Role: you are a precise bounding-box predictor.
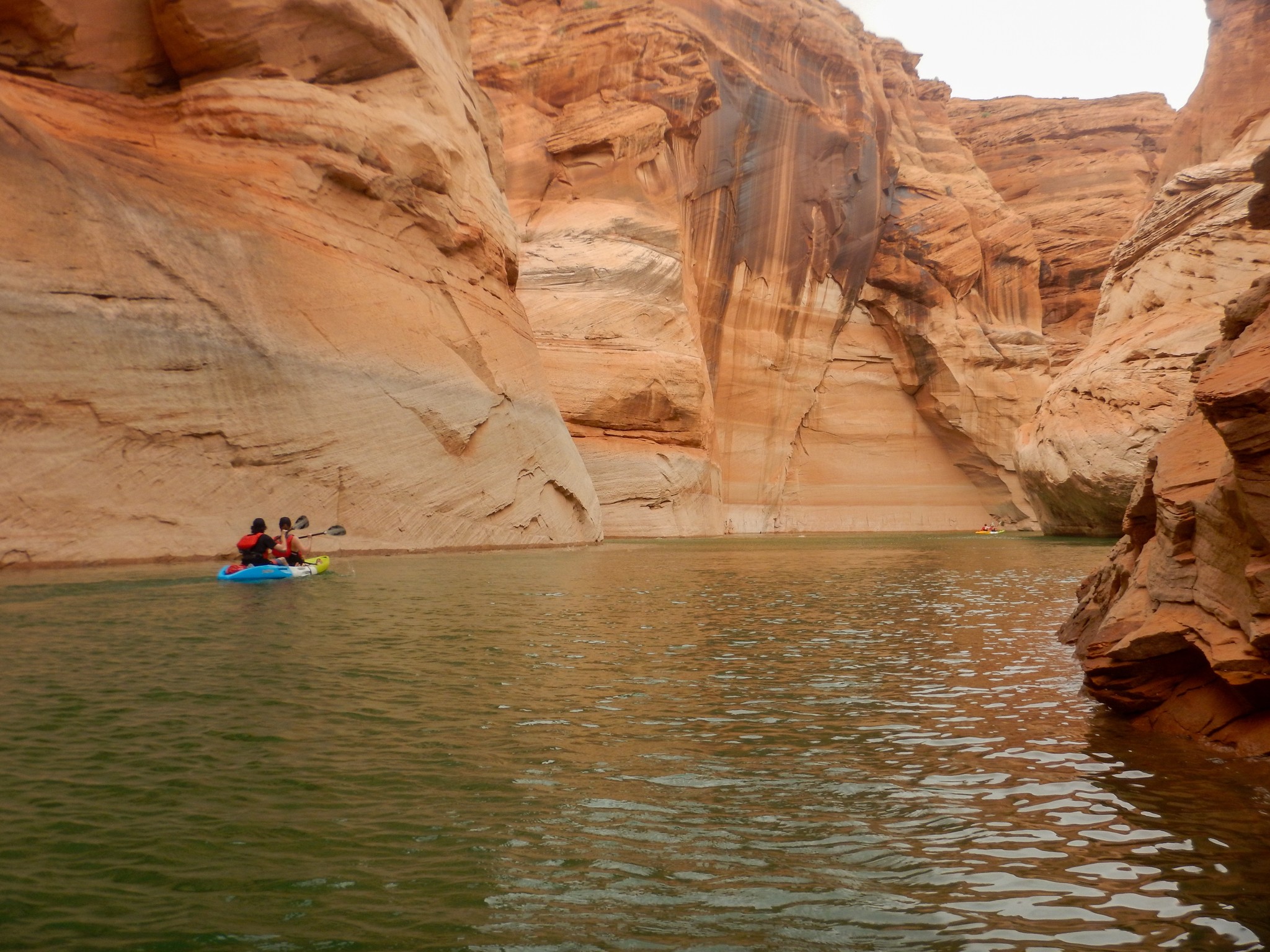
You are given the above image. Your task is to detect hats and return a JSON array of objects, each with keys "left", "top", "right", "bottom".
[
  {"left": 253, "top": 518, "right": 264, "bottom": 527},
  {"left": 280, "top": 517, "right": 291, "bottom": 527}
]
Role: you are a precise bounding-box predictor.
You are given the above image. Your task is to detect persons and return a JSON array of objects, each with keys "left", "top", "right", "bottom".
[
  {"left": 989, "top": 524, "right": 998, "bottom": 532},
  {"left": 981, "top": 524, "right": 991, "bottom": 531},
  {"left": 236, "top": 518, "right": 289, "bottom": 568},
  {"left": 271, "top": 517, "right": 323, "bottom": 567}
]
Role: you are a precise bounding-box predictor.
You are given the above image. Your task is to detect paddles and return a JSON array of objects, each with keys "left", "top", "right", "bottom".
[
  {"left": 298, "top": 525, "right": 346, "bottom": 539},
  {"left": 273, "top": 515, "right": 309, "bottom": 541}
]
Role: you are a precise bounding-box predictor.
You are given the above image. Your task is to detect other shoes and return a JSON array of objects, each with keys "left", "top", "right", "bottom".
[{"left": 316, "top": 558, "right": 323, "bottom": 565}]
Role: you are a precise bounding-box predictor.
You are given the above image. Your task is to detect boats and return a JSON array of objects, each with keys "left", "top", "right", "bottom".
[
  {"left": 214, "top": 553, "right": 336, "bottom": 583},
  {"left": 975, "top": 530, "right": 1005, "bottom": 534}
]
